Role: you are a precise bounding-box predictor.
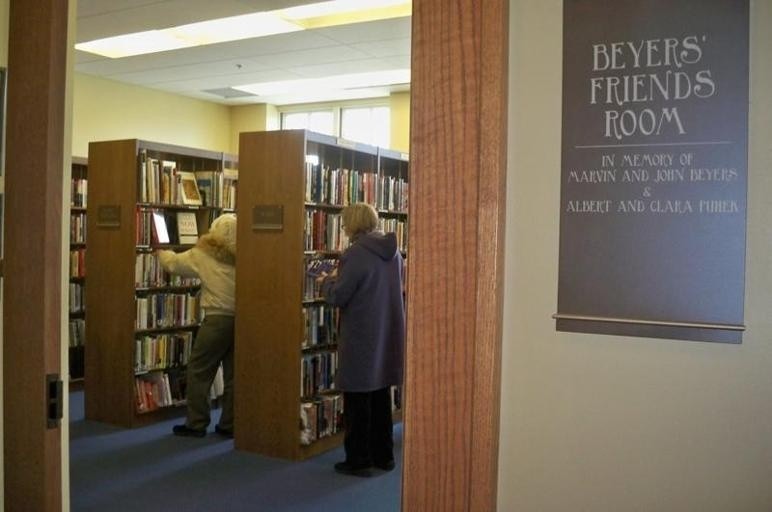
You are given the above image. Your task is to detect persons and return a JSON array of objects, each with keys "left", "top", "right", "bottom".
[
  {"left": 315, "top": 204, "right": 406, "bottom": 473},
  {"left": 155, "top": 213, "right": 238, "bottom": 435}
]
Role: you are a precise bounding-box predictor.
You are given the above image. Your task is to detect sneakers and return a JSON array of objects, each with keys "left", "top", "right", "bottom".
[
  {"left": 215, "top": 424, "right": 233, "bottom": 438},
  {"left": 173, "top": 425, "right": 206, "bottom": 437},
  {"left": 335, "top": 461, "right": 395, "bottom": 477}
]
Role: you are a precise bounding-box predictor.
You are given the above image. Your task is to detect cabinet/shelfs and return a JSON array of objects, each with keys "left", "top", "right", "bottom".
[
  {"left": 69, "top": 156, "right": 88, "bottom": 392},
  {"left": 85, "top": 138, "right": 238, "bottom": 430},
  {"left": 238, "top": 130, "right": 409, "bottom": 461}
]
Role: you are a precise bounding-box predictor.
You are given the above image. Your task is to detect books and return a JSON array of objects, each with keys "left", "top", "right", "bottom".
[
  {"left": 134, "top": 148, "right": 238, "bottom": 411},
  {"left": 306, "top": 163, "right": 409, "bottom": 444},
  {"left": 70, "top": 180, "right": 88, "bottom": 347}
]
